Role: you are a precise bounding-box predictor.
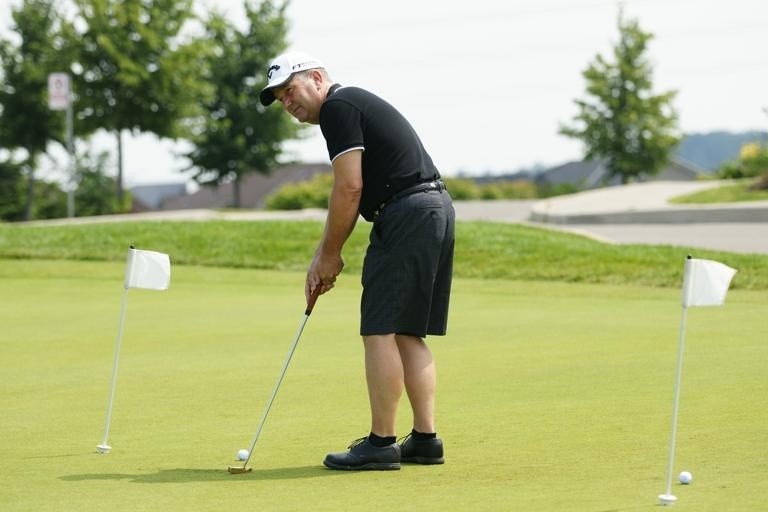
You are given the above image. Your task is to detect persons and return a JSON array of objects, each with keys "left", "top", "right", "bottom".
[{"left": 260, "top": 52, "right": 455, "bottom": 470}]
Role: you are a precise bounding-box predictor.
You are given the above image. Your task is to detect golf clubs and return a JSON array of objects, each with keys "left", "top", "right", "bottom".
[{"left": 228, "top": 275, "right": 323, "bottom": 473}]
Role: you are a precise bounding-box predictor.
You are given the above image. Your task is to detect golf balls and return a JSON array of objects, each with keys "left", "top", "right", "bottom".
[
  {"left": 680, "top": 471, "right": 691, "bottom": 483},
  {"left": 237, "top": 449, "right": 248, "bottom": 460}
]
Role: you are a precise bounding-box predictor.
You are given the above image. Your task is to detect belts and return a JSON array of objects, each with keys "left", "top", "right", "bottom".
[{"left": 398, "top": 182, "right": 445, "bottom": 197}]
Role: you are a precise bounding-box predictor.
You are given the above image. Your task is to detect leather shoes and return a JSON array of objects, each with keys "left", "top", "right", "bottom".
[{"left": 324, "top": 433, "right": 444, "bottom": 469}]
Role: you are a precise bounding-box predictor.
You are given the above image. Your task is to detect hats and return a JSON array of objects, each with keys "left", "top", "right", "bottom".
[{"left": 260, "top": 53, "right": 323, "bottom": 106}]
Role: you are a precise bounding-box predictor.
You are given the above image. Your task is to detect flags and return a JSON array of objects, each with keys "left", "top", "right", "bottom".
[
  {"left": 127, "top": 249, "right": 170, "bottom": 290},
  {"left": 684, "top": 260, "right": 738, "bottom": 307}
]
